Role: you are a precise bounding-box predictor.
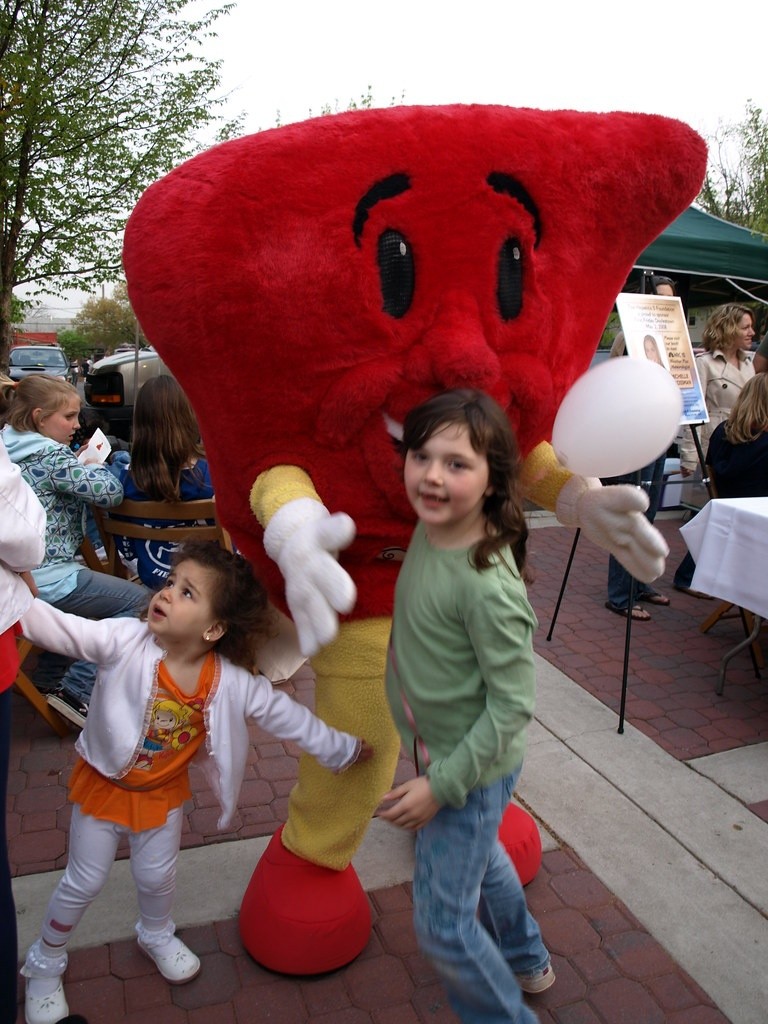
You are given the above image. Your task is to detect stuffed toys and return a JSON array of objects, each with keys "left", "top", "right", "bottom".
[{"left": 124, "top": 105, "right": 709, "bottom": 972}]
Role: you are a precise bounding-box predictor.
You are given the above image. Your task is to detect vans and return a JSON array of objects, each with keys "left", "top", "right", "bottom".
[{"left": 77, "top": 340, "right": 174, "bottom": 442}]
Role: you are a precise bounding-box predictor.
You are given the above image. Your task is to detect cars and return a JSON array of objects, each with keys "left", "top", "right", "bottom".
[{"left": 8, "top": 345, "right": 73, "bottom": 386}]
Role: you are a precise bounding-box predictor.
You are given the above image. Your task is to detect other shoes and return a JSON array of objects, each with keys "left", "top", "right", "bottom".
[
  {"left": 19, "top": 962, "right": 69, "bottom": 1024},
  {"left": 46, "top": 685, "right": 89, "bottom": 728},
  {"left": 137, "top": 932, "right": 201, "bottom": 984},
  {"left": 672, "top": 585, "right": 716, "bottom": 600},
  {"left": 514, "top": 960, "right": 555, "bottom": 993}
]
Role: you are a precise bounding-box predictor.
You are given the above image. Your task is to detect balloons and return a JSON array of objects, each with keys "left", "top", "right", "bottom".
[{"left": 551, "top": 356, "right": 684, "bottom": 478}]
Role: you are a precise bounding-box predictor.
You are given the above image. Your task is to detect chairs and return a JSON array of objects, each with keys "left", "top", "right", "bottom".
[
  {"left": 701, "top": 601, "right": 763, "bottom": 678},
  {"left": 20, "top": 356, "right": 31, "bottom": 362},
  {"left": 49, "top": 356, "right": 59, "bottom": 363},
  {"left": 12, "top": 497, "right": 234, "bottom": 739}
]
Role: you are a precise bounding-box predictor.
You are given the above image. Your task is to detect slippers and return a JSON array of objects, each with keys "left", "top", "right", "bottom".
[
  {"left": 605, "top": 600, "right": 651, "bottom": 621},
  {"left": 637, "top": 592, "right": 670, "bottom": 605}
]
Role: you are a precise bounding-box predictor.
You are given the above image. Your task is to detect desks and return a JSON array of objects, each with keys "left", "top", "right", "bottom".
[{"left": 675, "top": 497, "right": 768, "bottom": 702}]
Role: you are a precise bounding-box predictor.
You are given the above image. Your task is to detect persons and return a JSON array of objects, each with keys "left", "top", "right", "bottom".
[
  {"left": 0, "top": 435, "right": 86, "bottom": 1024},
  {"left": 19, "top": 541, "right": 373, "bottom": 1024},
  {"left": 604, "top": 276, "right": 675, "bottom": 619},
  {"left": 71, "top": 374, "right": 242, "bottom": 592},
  {"left": 378, "top": 388, "right": 556, "bottom": 1024},
  {"left": 704, "top": 332, "right": 768, "bottom": 498},
  {"left": 672, "top": 303, "right": 756, "bottom": 598},
  {"left": 0, "top": 373, "right": 151, "bottom": 729},
  {"left": 644, "top": 335, "right": 664, "bottom": 367},
  {"left": 72, "top": 358, "right": 93, "bottom": 385}
]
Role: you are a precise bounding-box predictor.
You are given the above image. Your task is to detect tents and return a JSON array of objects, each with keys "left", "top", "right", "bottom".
[{"left": 612, "top": 207, "right": 768, "bottom": 309}]
